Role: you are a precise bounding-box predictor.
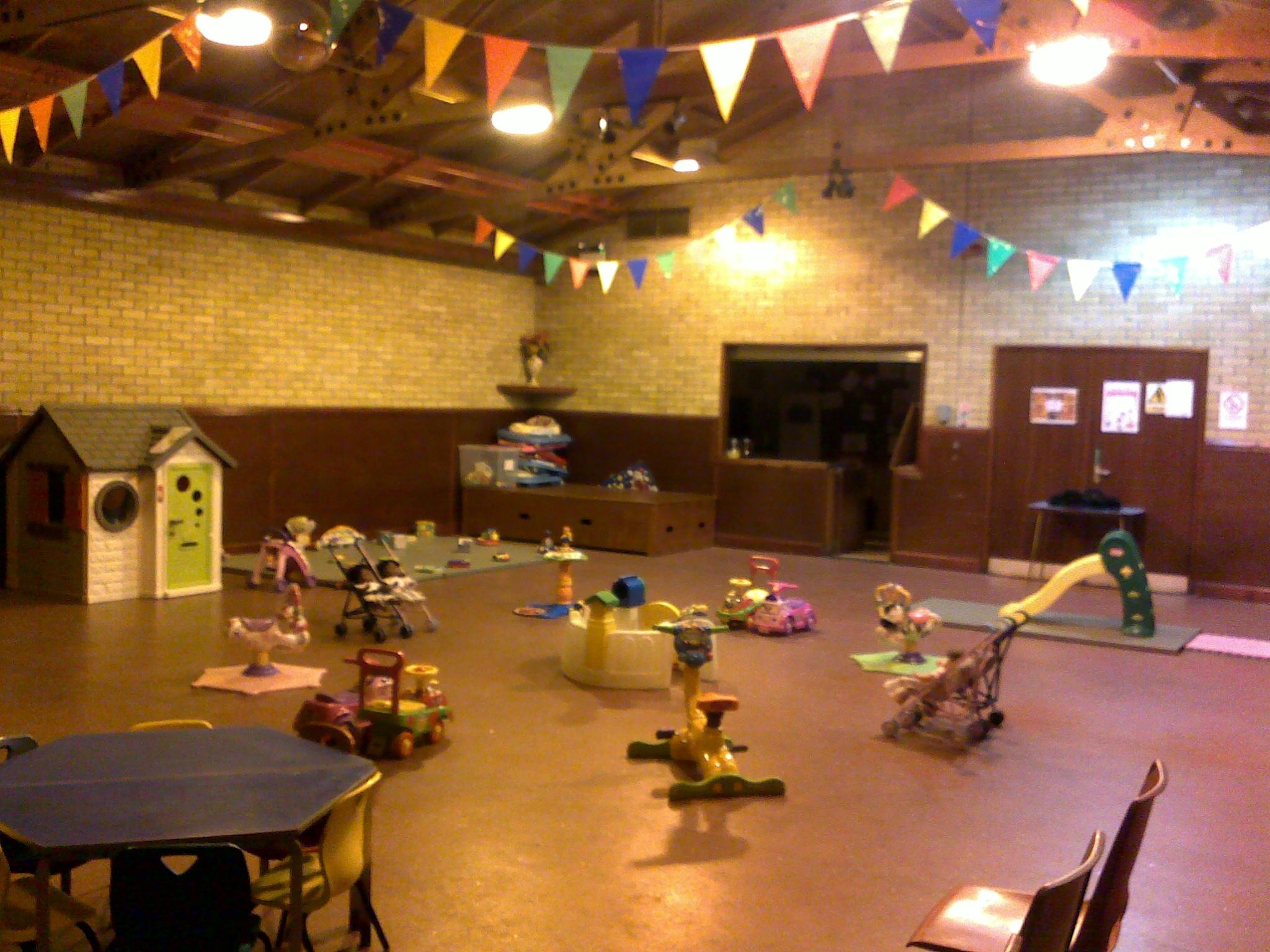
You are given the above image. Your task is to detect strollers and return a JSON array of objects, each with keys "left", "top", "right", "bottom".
[
  {"left": 879, "top": 611, "right": 1030, "bottom": 739},
  {"left": 328, "top": 529, "right": 439, "bottom": 643}
]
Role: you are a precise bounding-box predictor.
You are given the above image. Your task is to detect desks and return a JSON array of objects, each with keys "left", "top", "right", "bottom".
[{"left": 0, "top": 729, "right": 374, "bottom": 952}]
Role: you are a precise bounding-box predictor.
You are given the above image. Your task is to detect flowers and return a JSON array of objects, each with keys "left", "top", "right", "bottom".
[{"left": 520, "top": 328, "right": 553, "bottom": 361}]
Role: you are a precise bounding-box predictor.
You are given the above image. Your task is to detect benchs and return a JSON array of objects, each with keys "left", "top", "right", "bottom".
[
  {"left": 463, "top": 484, "right": 715, "bottom": 557},
  {"left": 1029, "top": 502, "right": 1146, "bottom": 556}
]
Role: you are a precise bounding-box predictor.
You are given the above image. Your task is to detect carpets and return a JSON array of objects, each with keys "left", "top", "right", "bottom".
[{"left": 223, "top": 536, "right": 547, "bottom": 582}]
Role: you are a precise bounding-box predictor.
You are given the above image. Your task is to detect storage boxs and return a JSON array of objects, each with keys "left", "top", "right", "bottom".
[
  {"left": 460, "top": 444, "right": 521, "bottom": 488},
  {"left": 561, "top": 620, "right": 674, "bottom": 690}
]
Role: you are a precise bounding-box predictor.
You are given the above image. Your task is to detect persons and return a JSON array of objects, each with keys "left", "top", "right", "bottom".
[
  {"left": 885, "top": 650, "right": 964, "bottom": 704},
  {"left": 338, "top": 560, "right": 423, "bottom": 603}
]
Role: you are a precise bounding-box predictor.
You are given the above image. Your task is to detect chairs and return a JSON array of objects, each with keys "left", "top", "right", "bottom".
[
  {"left": 906, "top": 759, "right": 1166, "bottom": 952},
  {"left": 0, "top": 720, "right": 390, "bottom": 952}
]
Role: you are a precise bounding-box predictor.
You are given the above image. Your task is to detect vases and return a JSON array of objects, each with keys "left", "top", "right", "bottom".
[{"left": 527, "top": 347, "right": 543, "bottom": 387}]
